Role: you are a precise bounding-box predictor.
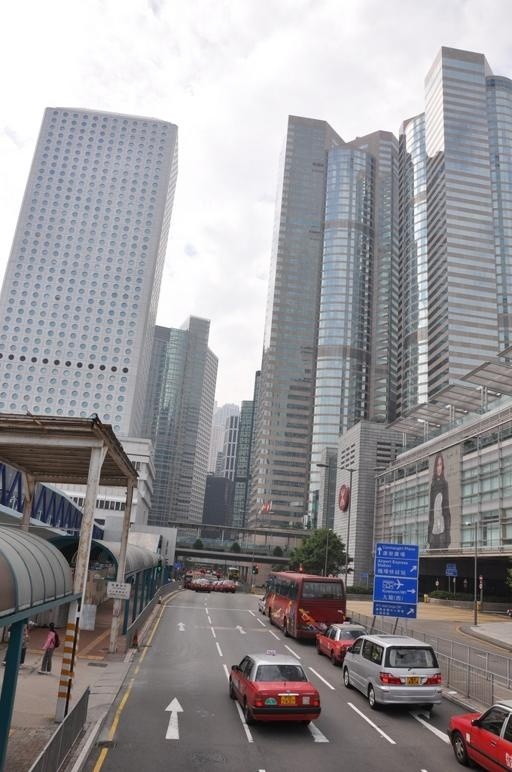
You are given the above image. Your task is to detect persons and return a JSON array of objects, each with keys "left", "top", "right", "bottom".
[
  {"left": 37, "top": 627, "right": 56, "bottom": 675},
  {"left": 19, "top": 628, "right": 29, "bottom": 668},
  {"left": 427, "top": 452, "right": 451, "bottom": 552}
]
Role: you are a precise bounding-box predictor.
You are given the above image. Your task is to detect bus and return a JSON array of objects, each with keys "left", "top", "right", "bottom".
[{"left": 264, "top": 570, "right": 347, "bottom": 641}]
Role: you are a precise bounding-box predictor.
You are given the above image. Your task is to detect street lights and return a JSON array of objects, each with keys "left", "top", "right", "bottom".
[{"left": 315, "top": 463, "right": 357, "bottom": 574}]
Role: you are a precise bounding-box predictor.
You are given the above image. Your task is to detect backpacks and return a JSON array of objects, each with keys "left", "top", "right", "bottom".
[{"left": 52, "top": 630, "right": 59, "bottom": 647}]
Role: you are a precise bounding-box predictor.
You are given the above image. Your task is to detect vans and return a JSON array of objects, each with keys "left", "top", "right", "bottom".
[{"left": 341, "top": 633, "right": 443, "bottom": 710}]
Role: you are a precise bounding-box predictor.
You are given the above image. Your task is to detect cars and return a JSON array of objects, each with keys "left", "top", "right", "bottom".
[
  {"left": 229, "top": 648, "right": 322, "bottom": 727},
  {"left": 446, "top": 699, "right": 512, "bottom": 772},
  {"left": 315, "top": 621, "right": 369, "bottom": 667}
]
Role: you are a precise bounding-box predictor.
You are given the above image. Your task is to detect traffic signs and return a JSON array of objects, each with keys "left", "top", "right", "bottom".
[{"left": 372, "top": 542, "right": 420, "bottom": 619}]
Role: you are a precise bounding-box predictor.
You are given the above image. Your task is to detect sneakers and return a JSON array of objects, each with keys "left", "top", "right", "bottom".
[{"left": 2, "top": 661, "right": 52, "bottom": 675}]
